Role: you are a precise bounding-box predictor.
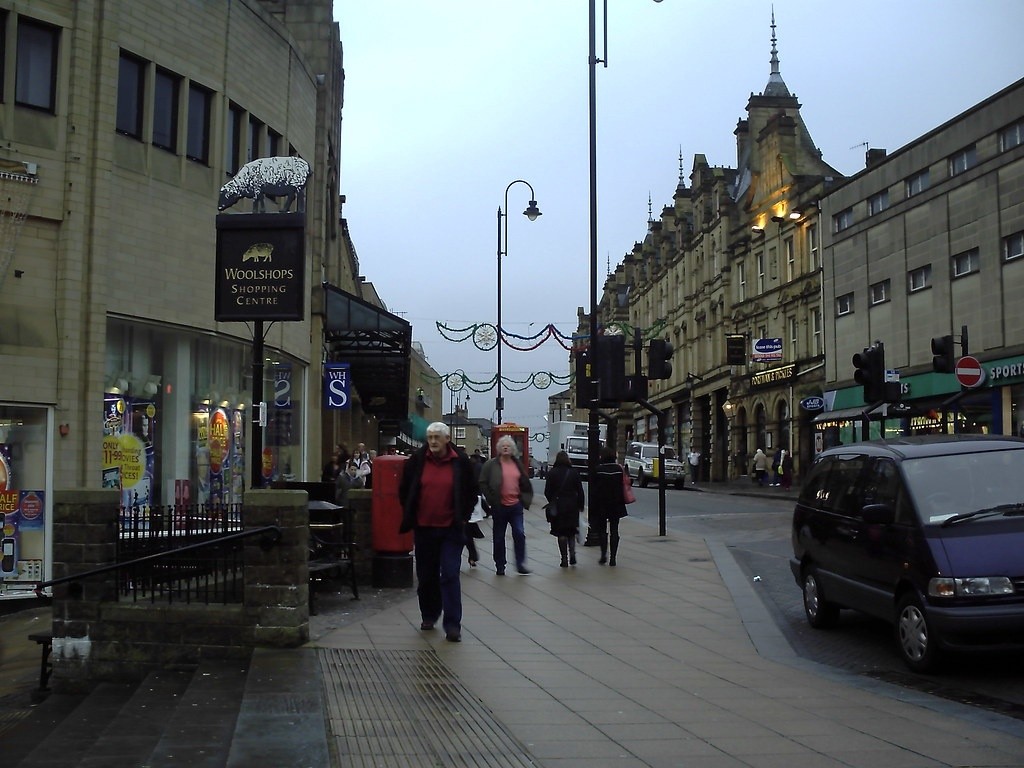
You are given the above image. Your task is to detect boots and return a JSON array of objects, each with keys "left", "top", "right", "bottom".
[
  {"left": 481, "top": 499, "right": 492, "bottom": 518},
  {"left": 559, "top": 539, "right": 568, "bottom": 567},
  {"left": 609, "top": 535, "right": 621, "bottom": 566},
  {"left": 598, "top": 532, "right": 608, "bottom": 564}
]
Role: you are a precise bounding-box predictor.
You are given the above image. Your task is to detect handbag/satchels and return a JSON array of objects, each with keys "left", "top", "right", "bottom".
[
  {"left": 752, "top": 463, "right": 756, "bottom": 473},
  {"left": 618, "top": 463, "right": 636, "bottom": 504},
  {"left": 542, "top": 502, "right": 558, "bottom": 523},
  {"left": 778, "top": 466, "right": 783, "bottom": 475}
]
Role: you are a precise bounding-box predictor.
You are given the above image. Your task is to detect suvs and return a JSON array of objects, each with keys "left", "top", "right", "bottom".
[{"left": 624, "top": 441, "right": 686, "bottom": 490}]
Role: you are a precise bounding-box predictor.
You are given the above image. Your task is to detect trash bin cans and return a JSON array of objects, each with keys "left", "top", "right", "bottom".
[
  {"left": 271, "top": 481, "right": 337, "bottom": 505},
  {"left": 369, "top": 455, "right": 414, "bottom": 588}
]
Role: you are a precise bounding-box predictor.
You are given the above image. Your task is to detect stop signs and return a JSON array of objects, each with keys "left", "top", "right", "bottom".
[{"left": 955, "top": 356, "right": 982, "bottom": 387}]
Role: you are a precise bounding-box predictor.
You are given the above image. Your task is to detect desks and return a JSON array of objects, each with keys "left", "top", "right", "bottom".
[{"left": 309, "top": 520, "right": 344, "bottom": 530}]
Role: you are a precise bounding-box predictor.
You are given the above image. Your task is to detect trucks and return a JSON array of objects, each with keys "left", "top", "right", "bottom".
[{"left": 547, "top": 420, "right": 607, "bottom": 476}]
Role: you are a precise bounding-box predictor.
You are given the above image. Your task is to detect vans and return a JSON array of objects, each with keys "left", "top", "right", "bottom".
[{"left": 789, "top": 433, "right": 1024, "bottom": 673}]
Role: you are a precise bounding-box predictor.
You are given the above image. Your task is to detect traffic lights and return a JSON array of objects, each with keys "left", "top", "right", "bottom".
[
  {"left": 852, "top": 353, "right": 869, "bottom": 384},
  {"left": 931, "top": 337, "right": 946, "bottom": 369},
  {"left": 664, "top": 342, "right": 674, "bottom": 379}
]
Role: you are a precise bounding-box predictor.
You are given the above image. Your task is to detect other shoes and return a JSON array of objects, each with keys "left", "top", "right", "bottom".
[
  {"left": 769, "top": 483, "right": 776, "bottom": 486},
  {"left": 568, "top": 536, "right": 576, "bottom": 564},
  {"left": 776, "top": 483, "right": 780, "bottom": 486},
  {"left": 496, "top": 568, "right": 505, "bottom": 575},
  {"left": 517, "top": 567, "right": 534, "bottom": 575},
  {"left": 420, "top": 619, "right": 436, "bottom": 629},
  {"left": 446, "top": 627, "right": 461, "bottom": 642}
]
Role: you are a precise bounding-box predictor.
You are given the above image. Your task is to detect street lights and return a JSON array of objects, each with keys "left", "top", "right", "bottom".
[
  {"left": 456, "top": 388, "right": 471, "bottom": 448},
  {"left": 496, "top": 179, "right": 543, "bottom": 426}
]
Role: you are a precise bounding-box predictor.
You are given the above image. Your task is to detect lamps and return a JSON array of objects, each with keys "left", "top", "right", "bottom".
[
  {"left": 142, "top": 374, "right": 162, "bottom": 396},
  {"left": 751, "top": 225, "right": 764, "bottom": 234},
  {"left": 415, "top": 384, "right": 425, "bottom": 400},
  {"left": 722, "top": 241, "right": 748, "bottom": 255},
  {"left": 771, "top": 215, "right": 785, "bottom": 223},
  {"left": 106, "top": 373, "right": 132, "bottom": 391},
  {"left": 725, "top": 402, "right": 734, "bottom": 410},
  {"left": 200, "top": 397, "right": 213, "bottom": 405},
  {"left": 789, "top": 209, "right": 804, "bottom": 220},
  {"left": 221, "top": 399, "right": 245, "bottom": 410}
]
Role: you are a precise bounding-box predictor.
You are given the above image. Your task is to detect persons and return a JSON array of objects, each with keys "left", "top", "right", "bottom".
[
  {"left": 688, "top": 446, "right": 701, "bottom": 485},
  {"left": 781, "top": 450, "right": 796, "bottom": 491},
  {"left": 399, "top": 421, "right": 478, "bottom": 641},
  {"left": 769, "top": 446, "right": 782, "bottom": 487},
  {"left": 539, "top": 451, "right": 585, "bottom": 568},
  {"left": 479, "top": 433, "right": 534, "bottom": 578},
  {"left": 592, "top": 446, "right": 635, "bottom": 567},
  {"left": 753, "top": 448, "right": 768, "bottom": 487},
  {"left": 328, "top": 431, "right": 497, "bottom": 527}
]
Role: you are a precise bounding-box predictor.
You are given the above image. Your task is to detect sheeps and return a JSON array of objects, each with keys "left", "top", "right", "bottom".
[{"left": 217, "top": 156, "right": 311, "bottom": 214}]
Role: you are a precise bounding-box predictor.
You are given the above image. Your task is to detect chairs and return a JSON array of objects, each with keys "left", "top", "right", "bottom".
[{"left": 311, "top": 504, "right": 361, "bottom": 617}]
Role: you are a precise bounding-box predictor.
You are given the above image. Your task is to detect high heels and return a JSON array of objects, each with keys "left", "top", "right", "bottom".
[{"left": 468, "top": 558, "right": 475, "bottom": 568}]
